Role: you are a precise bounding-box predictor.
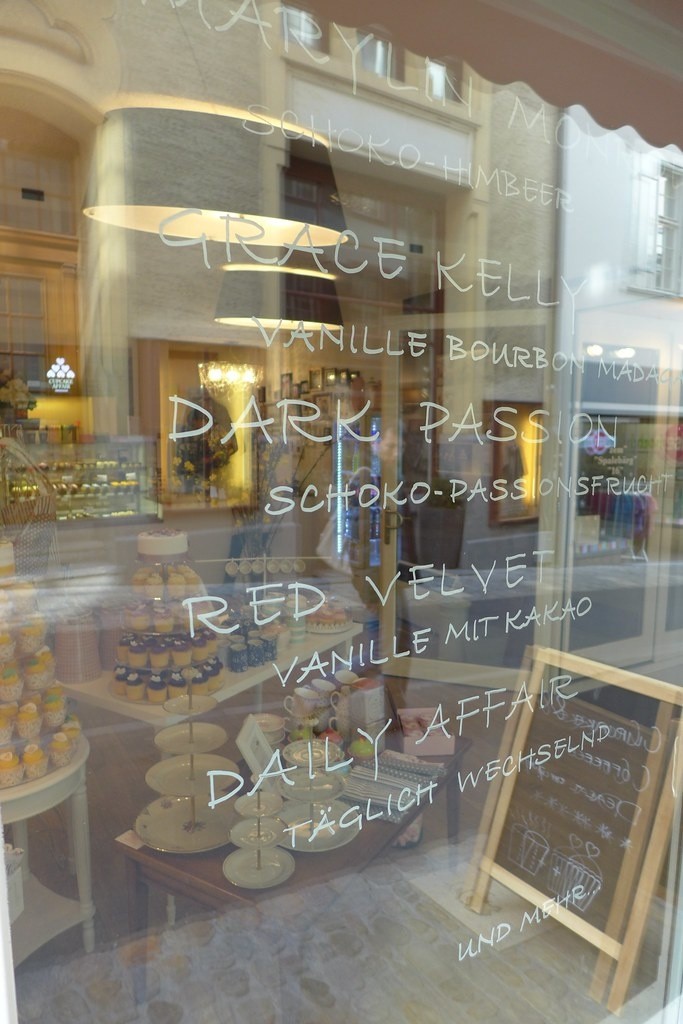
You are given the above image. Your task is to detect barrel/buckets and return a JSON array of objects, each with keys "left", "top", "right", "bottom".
[
  {"left": 244, "top": 713, "right": 284, "bottom": 755},
  {"left": 53, "top": 599, "right": 126, "bottom": 684}
]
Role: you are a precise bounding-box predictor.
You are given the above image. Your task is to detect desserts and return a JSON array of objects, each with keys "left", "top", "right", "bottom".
[
  {"left": 11, "top": 458, "right": 142, "bottom": 519},
  {"left": 0, "top": 542, "right": 81, "bottom": 787},
  {"left": 112, "top": 529, "right": 225, "bottom": 702}
]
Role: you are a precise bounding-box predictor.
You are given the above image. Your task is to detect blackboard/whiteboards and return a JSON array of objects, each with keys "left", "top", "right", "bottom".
[{"left": 484, "top": 685, "right": 654, "bottom": 938}]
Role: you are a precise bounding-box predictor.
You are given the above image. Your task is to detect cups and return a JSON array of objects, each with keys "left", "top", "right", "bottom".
[
  {"left": 261, "top": 592, "right": 306, "bottom": 644},
  {"left": 346, "top": 736, "right": 377, "bottom": 765},
  {"left": 284, "top": 669, "right": 359, "bottom": 733},
  {"left": 317, "top": 728, "right": 343, "bottom": 750},
  {"left": 286, "top": 724, "right": 315, "bottom": 745},
  {"left": 258, "top": 620, "right": 290, "bottom": 652},
  {"left": 224, "top": 630, "right": 278, "bottom": 672}
]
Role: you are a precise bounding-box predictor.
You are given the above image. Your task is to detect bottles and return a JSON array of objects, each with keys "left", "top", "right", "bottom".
[{"left": 344, "top": 421, "right": 380, "bottom": 558}]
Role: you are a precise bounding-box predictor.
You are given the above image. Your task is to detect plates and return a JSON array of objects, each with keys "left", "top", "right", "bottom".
[
  {"left": 234, "top": 791, "right": 283, "bottom": 818},
  {"left": 145, "top": 754, "right": 240, "bottom": 797},
  {"left": 271, "top": 800, "right": 360, "bottom": 853},
  {"left": 222, "top": 847, "right": 296, "bottom": 889},
  {"left": 163, "top": 695, "right": 219, "bottom": 717},
  {"left": 226, "top": 818, "right": 290, "bottom": 851},
  {"left": 277, "top": 768, "right": 346, "bottom": 805},
  {"left": 153, "top": 722, "right": 228, "bottom": 756},
  {"left": 134, "top": 791, "right": 243, "bottom": 853},
  {"left": 282, "top": 739, "right": 342, "bottom": 767}
]
